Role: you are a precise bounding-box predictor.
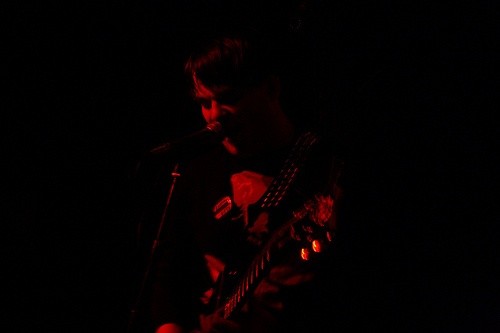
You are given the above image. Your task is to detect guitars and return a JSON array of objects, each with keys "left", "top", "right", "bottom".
[{"left": 223, "top": 195, "right": 333, "bottom": 320}]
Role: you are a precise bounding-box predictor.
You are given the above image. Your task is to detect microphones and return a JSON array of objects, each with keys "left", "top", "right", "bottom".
[{"left": 152, "top": 121, "right": 224, "bottom": 152}]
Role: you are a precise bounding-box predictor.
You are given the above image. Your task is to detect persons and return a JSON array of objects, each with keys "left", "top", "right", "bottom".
[{"left": 146, "top": 39, "right": 372, "bottom": 333}]
